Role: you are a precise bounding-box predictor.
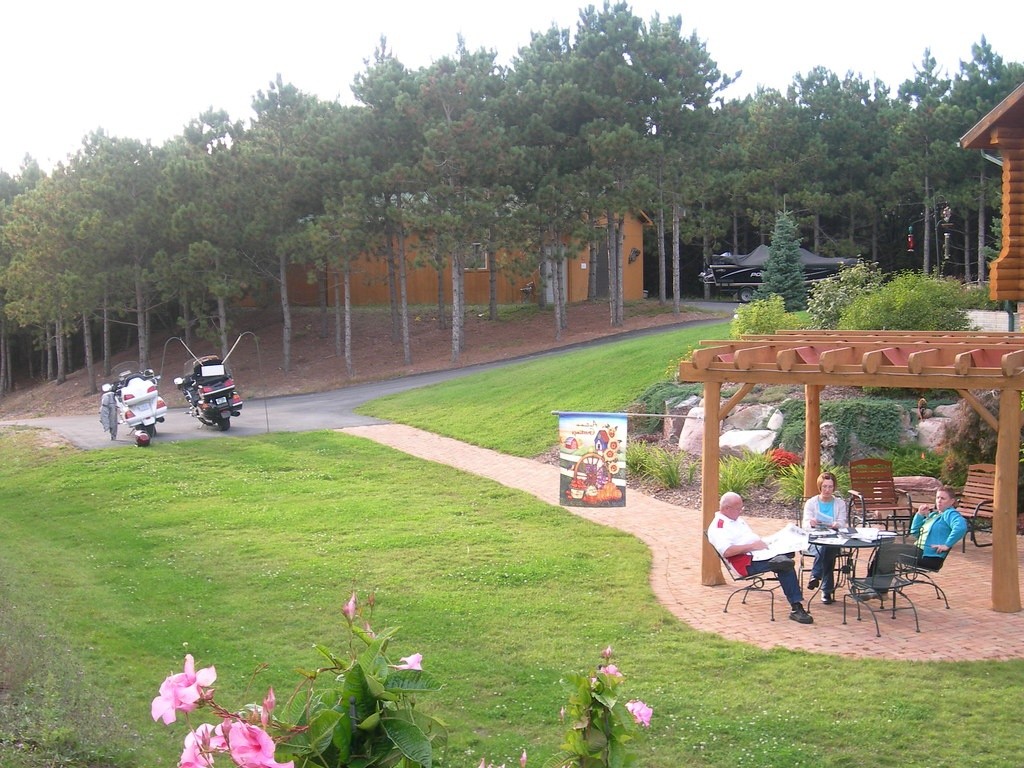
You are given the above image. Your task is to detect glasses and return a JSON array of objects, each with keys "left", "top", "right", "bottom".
[{"left": 729, "top": 505, "right": 746, "bottom": 511}]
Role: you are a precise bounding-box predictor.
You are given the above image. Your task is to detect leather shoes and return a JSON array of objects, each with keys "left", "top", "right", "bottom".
[
  {"left": 789, "top": 604, "right": 814, "bottom": 623},
  {"left": 768, "top": 554, "right": 795, "bottom": 573}
]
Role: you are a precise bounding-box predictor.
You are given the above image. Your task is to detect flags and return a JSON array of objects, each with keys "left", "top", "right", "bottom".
[{"left": 559, "top": 413, "right": 626, "bottom": 508}]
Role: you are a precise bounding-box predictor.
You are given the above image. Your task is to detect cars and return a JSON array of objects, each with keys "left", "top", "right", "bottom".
[{"left": 698, "top": 243, "right": 867, "bottom": 303}]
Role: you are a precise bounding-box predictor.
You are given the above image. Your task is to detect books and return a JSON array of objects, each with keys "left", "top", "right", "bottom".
[{"left": 846, "top": 528, "right": 858, "bottom": 536}]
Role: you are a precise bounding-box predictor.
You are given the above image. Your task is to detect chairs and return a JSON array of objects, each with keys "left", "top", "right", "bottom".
[
  {"left": 848, "top": 458, "right": 912, "bottom": 538},
  {"left": 953, "top": 463, "right": 995, "bottom": 553},
  {"left": 842, "top": 527, "right": 924, "bottom": 636},
  {"left": 794, "top": 493, "right": 851, "bottom": 597},
  {"left": 703, "top": 529, "right": 782, "bottom": 621},
  {"left": 895, "top": 544, "right": 955, "bottom": 609}
]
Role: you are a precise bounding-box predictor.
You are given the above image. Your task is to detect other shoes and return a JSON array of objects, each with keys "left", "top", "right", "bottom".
[
  {"left": 851, "top": 582, "right": 873, "bottom": 590},
  {"left": 856, "top": 588, "right": 888, "bottom": 601},
  {"left": 807, "top": 575, "right": 819, "bottom": 590},
  {"left": 821, "top": 590, "right": 832, "bottom": 604}
]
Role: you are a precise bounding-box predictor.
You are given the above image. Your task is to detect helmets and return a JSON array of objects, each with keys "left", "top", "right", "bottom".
[{"left": 134, "top": 429, "right": 151, "bottom": 447}]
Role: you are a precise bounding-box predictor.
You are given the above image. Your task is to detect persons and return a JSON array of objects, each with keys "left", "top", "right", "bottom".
[
  {"left": 707, "top": 491, "right": 814, "bottom": 624},
  {"left": 802, "top": 472, "right": 846, "bottom": 604},
  {"left": 859, "top": 485, "right": 967, "bottom": 601}
]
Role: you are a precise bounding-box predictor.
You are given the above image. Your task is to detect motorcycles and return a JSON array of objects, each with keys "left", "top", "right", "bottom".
[
  {"left": 174, "top": 355, "right": 243, "bottom": 432},
  {"left": 102, "top": 361, "right": 168, "bottom": 438}
]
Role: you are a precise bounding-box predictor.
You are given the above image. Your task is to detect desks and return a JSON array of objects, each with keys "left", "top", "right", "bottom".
[{"left": 803, "top": 528, "right": 896, "bottom": 612}]
